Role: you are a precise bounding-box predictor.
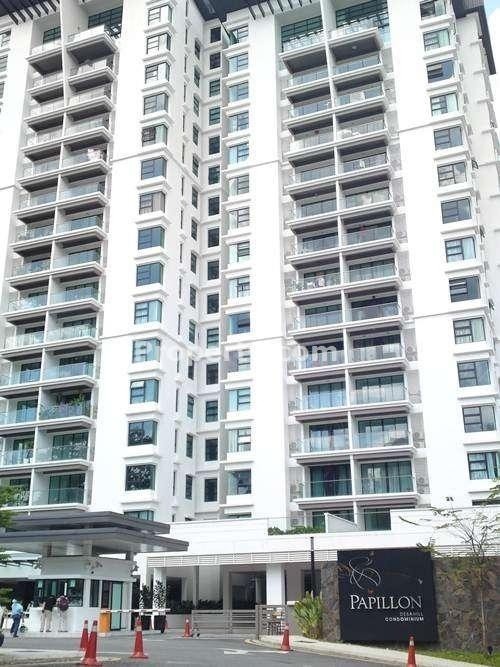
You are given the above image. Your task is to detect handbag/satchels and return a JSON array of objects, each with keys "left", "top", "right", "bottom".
[
  {"left": 61, "top": 604, "right": 68, "bottom": 611},
  {"left": 20, "top": 624, "right": 25, "bottom": 632}
]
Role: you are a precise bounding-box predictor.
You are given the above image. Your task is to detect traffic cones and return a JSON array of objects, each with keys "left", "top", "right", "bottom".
[
  {"left": 406, "top": 636, "right": 418, "bottom": 667},
  {"left": 279, "top": 623, "right": 294, "bottom": 651},
  {"left": 78, "top": 620, "right": 104, "bottom": 667},
  {"left": 181, "top": 618, "right": 192, "bottom": 637},
  {"left": 128, "top": 618, "right": 148, "bottom": 658}
]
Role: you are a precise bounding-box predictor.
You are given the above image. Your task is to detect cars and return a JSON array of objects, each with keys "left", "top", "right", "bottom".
[{"left": 3, "top": 612, "right": 29, "bottom": 633}]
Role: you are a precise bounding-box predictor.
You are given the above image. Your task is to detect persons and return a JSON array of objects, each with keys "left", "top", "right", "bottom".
[
  {"left": 10, "top": 598, "right": 24, "bottom": 640},
  {"left": 39, "top": 592, "right": 71, "bottom": 633}
]
